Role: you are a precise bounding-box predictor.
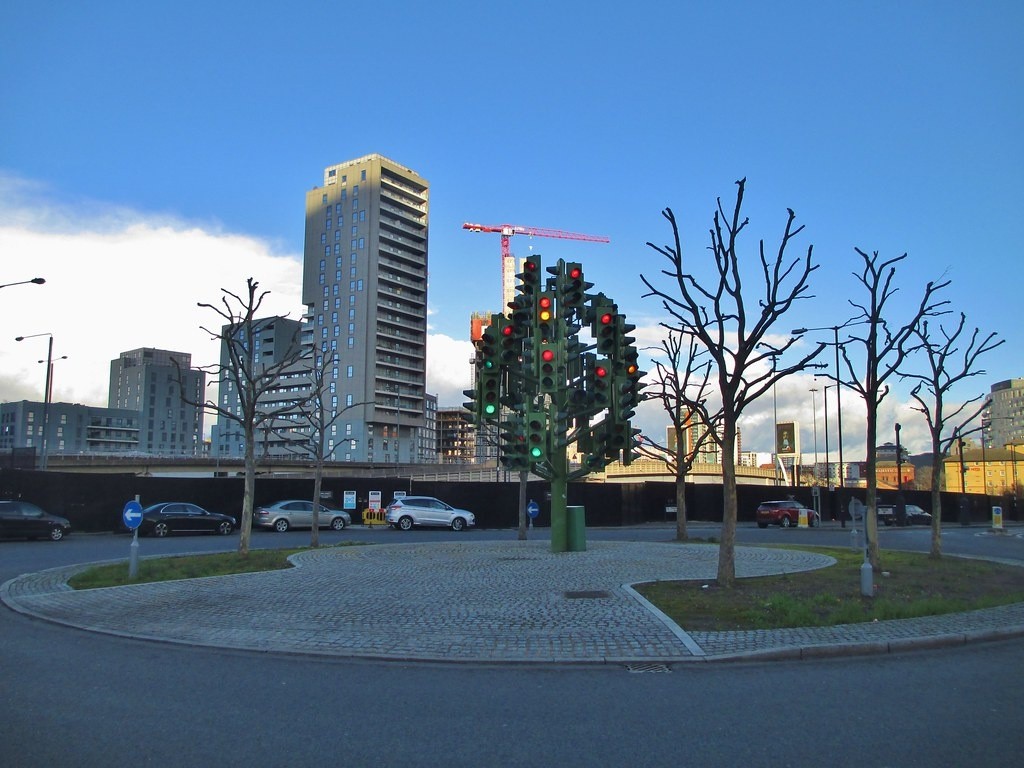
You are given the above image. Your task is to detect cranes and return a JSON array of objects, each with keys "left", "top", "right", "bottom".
[{"left": 461, "top": 221, "right": 610, "bottom": 318}]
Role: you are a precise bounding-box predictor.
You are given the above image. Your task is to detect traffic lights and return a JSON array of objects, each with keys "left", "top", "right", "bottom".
[
  {"left": 595, "top": 306, "right": 614, "bottom": 355},
  {"left": 482, "top": 370, "right": 499, "bottom": 420},
  {"left": 538, "top": 343, "right": 559, "bottom": 393},
  {"left": 613, "top": 313, "right": 637, "bottom": 364},
  {"left": 594, "top": 359, "right": 613, "bottom": 408},
  {"left": 507, "top": 253, "right": 541, "bottom": 328},
  {"left": 481, "top": 327, "right": 500, "bottom": 373},
  {"left": 500, "top": 395, "right": 531, "bottom": 471},
  {"left": 528, "top": 412, "right": 547, "bottom": 463},
  {"left": 622, "top": 420, "right": 642, "bottom": 467},
  {"left": 623, "top": 351, "right": 648, "bottom": 408},
  {"left": 581, "top": 414, "right": 629, "bottom": 473},
  {"left": 520, "top": 327, "right": 543, "bottom": 378},
  {"left": 565, "top": 262, "right": 583, "bottom": 307},
  {"left": 615, "top": 375, "right": 636, "bottom": 424},
  {"left": 576, "top": 272, "right": 596, "bottom": 319},
  {"left": 545, "top": 258, "right": 578, "bottom": 319},
  {"left": 462, "top": 381, "right": 483, "bottom": 429},
  {"left": 567, "top": 387, "right": 588, "bottom": 407},
  {"left": 550, "top": 403, "right": 570, "bottom": 455},
  {"left": 536, "top": 291, "right": 554, "bottom": 343},
  {"left": 599, "top": 297, "right": 618, "bottom": 312},
  {"left": 567, "top": 335, "right": 588, "bottom": 380},
  {"left": 498, "top": 318, "right": 516, "bottom": 365},
  {"left": 557, "top": 316, "right": 579, "bottom": 369}
]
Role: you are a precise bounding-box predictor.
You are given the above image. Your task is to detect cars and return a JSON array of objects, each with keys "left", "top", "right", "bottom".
[
  {"left": 0, "top": 501, "right": 72, "bottom": 541},
  {"left": 883, "top": 504, "right": 933, "bottom": 527},
  {"left": 252, "top": 500, "right": 352, "bottom": 532},
  {"left": 119, "top": 501, "right": 237, "bottom": 539}
]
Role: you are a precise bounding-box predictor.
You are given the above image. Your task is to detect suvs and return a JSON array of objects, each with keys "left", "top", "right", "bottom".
[
  {"left": 384, "top": 496, "right": 476, "bottom": 531},
  {"left": 756, "top": 501, "right": 820, "bottom": 529}
]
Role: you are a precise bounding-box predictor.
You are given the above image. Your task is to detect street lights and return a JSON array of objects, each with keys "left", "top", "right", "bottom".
[
  {"left": 15, "top": 332, "right": 68, "bottom": 470},
  {"left": 791, "top": 317, "right": 886, "bottom": 528},
  {"left": 808, "top": 388, "right": 818, "bottom": 528},
  {"left": 824, "top": 379, "right": 856, "bottom": 522}
]
